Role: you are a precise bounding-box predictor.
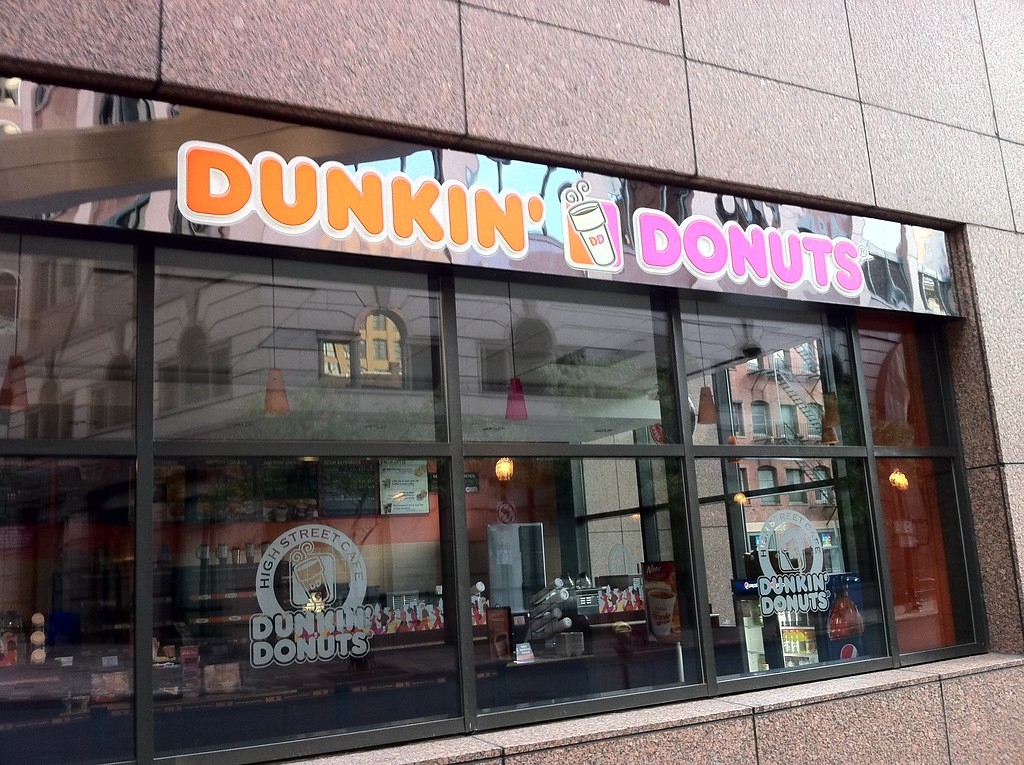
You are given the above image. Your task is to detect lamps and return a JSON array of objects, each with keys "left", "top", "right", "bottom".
[
  {"left": 695, "top": 300, "right": 719, "bottom": 424},
  {"left": 726, "top": 369, "right": 744, "bottom": 464},
  {"left": 504, "top": 282, "right": 528, "bottom": 422},
  {"left": 819, "top": 313, "right": 842, "bottom": 445},
  {"left": 0, "top": 235, "right": 29, "bottom": 410},
  {"left": 263, "top": 259, "right": 290, "bottom": 418}
]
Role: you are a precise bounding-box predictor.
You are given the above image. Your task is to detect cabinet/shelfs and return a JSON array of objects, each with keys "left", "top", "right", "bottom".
[{"left": 54, "top": 560, "right": 293, "bottom": 650}]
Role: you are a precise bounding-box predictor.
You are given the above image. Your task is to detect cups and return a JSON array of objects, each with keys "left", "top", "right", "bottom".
[
  {"left": 194, "top": 540, "right": 274, "bottom": 568},
  {"left": 647, "top": 589, "right": 676, "bottom": 638},
  {"left": 28, "top": 613, "right": 46, "bottom": 664}
]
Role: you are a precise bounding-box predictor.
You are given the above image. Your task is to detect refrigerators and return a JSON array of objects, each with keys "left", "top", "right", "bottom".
[{"left": 729, "top": 570, "right": 874, "bottom": 676}]
